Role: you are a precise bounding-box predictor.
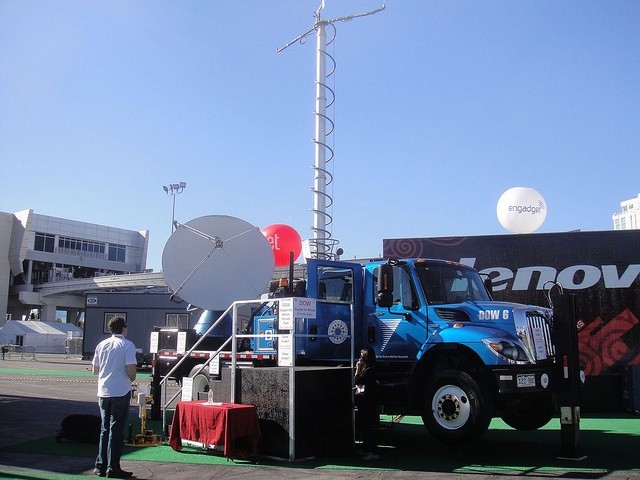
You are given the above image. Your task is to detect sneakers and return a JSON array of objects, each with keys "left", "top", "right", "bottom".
[{"left": 363, "top": 453, "right": 379, "bottom": 461}]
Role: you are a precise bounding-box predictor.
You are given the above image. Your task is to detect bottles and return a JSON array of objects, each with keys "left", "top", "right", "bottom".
[{"left": 208, "top": 388, "right": 213, "bottom": 405}]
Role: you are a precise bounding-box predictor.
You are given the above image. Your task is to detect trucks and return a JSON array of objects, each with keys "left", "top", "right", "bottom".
[{"left": 150, "top": 257, "right": 553, "bottom": 443}]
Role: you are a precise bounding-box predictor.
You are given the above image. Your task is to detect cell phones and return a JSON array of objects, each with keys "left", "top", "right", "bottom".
[{"left": 360, "top": 358, "right": 363, "bottom": 362}]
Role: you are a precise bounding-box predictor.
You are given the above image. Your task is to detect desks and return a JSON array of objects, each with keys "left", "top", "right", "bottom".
[{"left": 168, "top": 401, "right": 258, "bottom": 462}]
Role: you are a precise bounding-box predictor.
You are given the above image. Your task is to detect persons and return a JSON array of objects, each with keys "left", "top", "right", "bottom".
[
  {"left": 91, "top": 316, "right": 139, "bottom": 478},
  {"left": 354, "top": 347, "right": 390, "bottom": 460}
]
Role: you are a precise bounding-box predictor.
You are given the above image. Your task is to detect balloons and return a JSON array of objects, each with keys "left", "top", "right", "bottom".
[
  {"left": 496, "top": 186, "right": 547, "bottom": 235},
  {"left": 261, "top": 223, "right": 303, "bottom": 269}
]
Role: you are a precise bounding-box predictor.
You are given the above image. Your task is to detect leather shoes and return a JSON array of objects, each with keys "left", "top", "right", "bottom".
[
  {"left": 107, "top": 468, "right": 134, "bottom": 479},
  {"left": 94, "top": 467, "right": 106, "bottom": 475}
]
